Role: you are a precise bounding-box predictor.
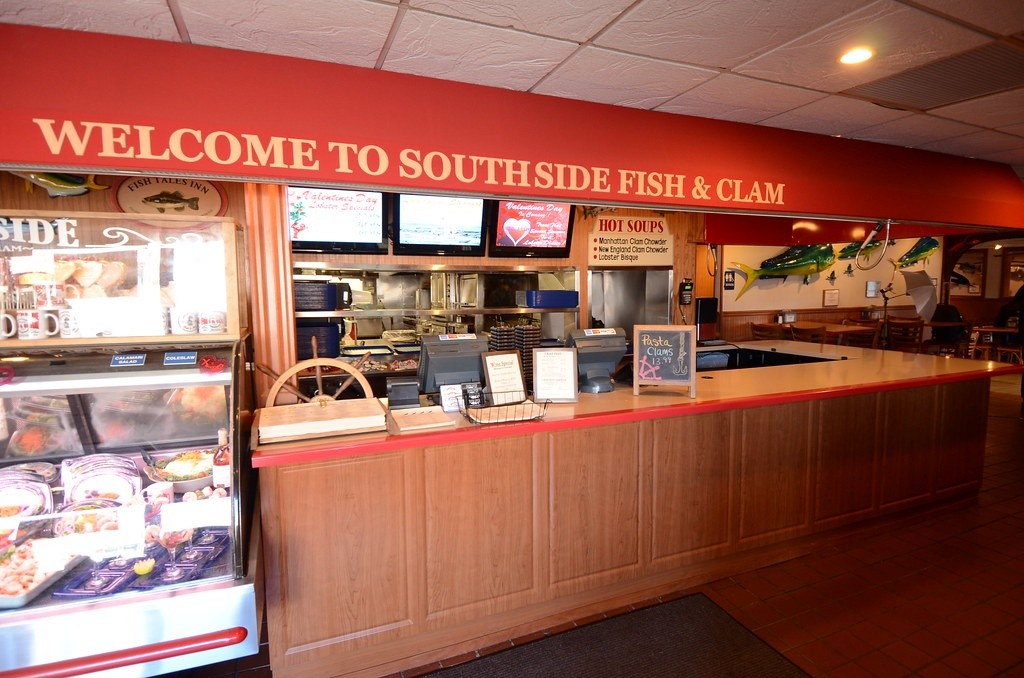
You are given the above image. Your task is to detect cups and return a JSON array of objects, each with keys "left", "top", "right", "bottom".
[
  {"left": 161, "top": 307, "right": 227, "bottom": 333},
  {"left": 0, "top": 309, "right": 18, "bottom": 341},
  {"left": 16, "top": 309, "right": 60, "bottom": 340}
]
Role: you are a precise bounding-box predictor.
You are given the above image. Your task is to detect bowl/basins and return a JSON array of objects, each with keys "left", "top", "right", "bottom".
[{"left": 147, "top": 467, "right": 213, "bottom": 493}]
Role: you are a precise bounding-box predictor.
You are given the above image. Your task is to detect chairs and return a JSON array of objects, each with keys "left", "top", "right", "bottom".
[
  {"left": 941, "top": 332, "right": 1023, "bottom": 365},
  {"left": 886, "top": 315, "right": 923, "bottom": 352},
  {"left": 751, "top": 318, "right": 885, "bottom": 346}
]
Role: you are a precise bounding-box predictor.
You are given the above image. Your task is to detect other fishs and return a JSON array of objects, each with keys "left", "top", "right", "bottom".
[
  {"left": 834, "top": 240, "right": 883, "bottom": 260},
  {"left": 8, "top": 172, "right": 109, "bottom": 198},
  {"left": 888, "top": 236, "right": 942, "bottom": 280},
  {"left": 730, "top": 241, "right": 836, "bottom": 301}
]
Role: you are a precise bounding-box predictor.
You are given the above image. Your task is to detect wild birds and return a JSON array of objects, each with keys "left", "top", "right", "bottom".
[
  {"left": 845, "top": 262, "right": 853, "bottom": 274},
  {"left": 826, "top": 271, "right": 837, "bottom": 281}
]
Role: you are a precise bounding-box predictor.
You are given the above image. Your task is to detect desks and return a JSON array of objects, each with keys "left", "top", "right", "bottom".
[
  {"left": 924, "top": 321, "right": 966, "bottom": 327},
  {"left": 784, "top": 320, "right": 877, "bottom": 335},
  {"left": 974, "top": 326, "right": 1018, "bottom": 332}
]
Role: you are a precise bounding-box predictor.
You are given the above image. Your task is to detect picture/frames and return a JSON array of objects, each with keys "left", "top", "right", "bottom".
[
  {"left": 949, "top": 248, "right": 988, "bottom": 298},
  {"left": 998, "top": 247, "right": 1024, "bottom": 298}
]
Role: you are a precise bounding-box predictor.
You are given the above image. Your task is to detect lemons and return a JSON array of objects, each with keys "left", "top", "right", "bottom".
[{"left": 134, "top": 558, "right": 155, "bottom": 575}]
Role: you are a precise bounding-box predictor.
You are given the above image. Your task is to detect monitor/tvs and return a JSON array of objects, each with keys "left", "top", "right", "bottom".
[
  {"left": 417, "top": 334, "right": 488, "bottom": 394},
  {"left": 286, "top": 185, "right": 388, "bottom": 255},
  {"left": 562, "top": 326, "right": 627, "bottom": 392},
  {"left": 392, "top": 194, "right": 487, "bottom": 257},
  {"left": 488, "top": 200, "right": 577, "bottom": 258}
]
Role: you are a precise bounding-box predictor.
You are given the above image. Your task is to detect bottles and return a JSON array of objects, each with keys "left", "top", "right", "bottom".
[{"left": 212, "top": 428, "right": 232, "bottom": 495}]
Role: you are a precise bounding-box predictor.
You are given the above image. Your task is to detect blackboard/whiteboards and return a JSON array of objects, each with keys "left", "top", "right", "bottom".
[{"left": 633, "top": 325, "right": 696, "bottom": 386}]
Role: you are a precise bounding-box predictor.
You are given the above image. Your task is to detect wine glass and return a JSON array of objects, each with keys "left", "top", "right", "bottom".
[{"left": 152, "top": 515, "right": 205, "bottom": 581}]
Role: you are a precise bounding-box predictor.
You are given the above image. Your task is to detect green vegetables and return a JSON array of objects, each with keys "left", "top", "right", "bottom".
[{"left": 154, "top": 453, "right": 212, "bottom": 481}]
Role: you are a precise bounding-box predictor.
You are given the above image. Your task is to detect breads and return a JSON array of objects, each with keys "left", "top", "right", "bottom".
[{"left": 18, "top": 258, "right": 130, "bottom": 292}]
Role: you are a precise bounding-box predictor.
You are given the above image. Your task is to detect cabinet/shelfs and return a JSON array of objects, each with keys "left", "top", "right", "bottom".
[{"left": 295, "top": 307, "right": 581, "bottom": 380}]
